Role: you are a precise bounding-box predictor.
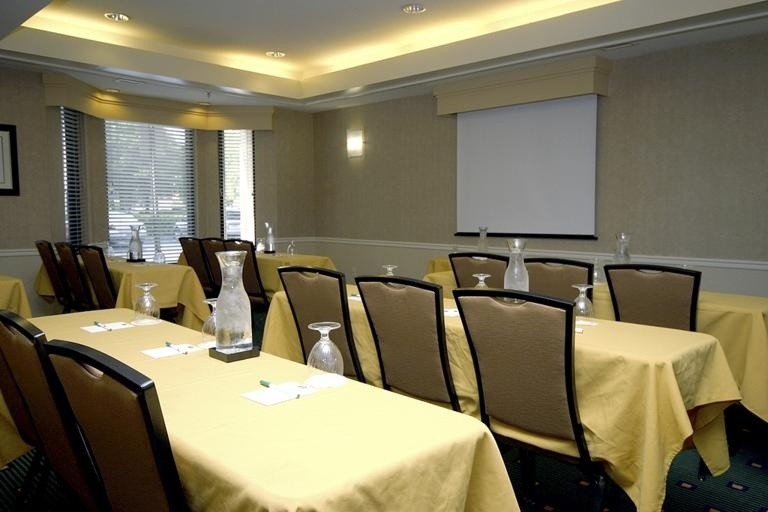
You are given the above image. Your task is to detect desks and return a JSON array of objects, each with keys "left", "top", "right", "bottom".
[
  {"left": 33, "top": 257, "right": 212, "bottom": 334},
  {"left": 22, "top": 307, "right": 522, "bottom": 511},
  {"left": 177, "top": 251, "right": 337, "bottom": 302},
  {"left": 260, "top": 281, "right": 742, "bottom": 512},
  {"left": 421, "top": 269, "right": 768, "bottom": 423}
]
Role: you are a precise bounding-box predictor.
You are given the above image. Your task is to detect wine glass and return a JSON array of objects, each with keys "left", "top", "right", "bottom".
[
  {"left": 286, "top": 239, "right": 296, "bottom": 256},
  {"left": 306, "top": 321, "right": 344, "bottom": 386},
  {"left": 153, "top": 246, "right": 167, "bottom": 267},
  {"left": 134, "top": 283, "right": 160, "bottom": 323},
  {"left": 571, "top": 282, "right": 594, "bottom": 328},
  {"left": 473, "top": 273, "right": 491, "bottom": 289},
  {"left": 103, "top": 238, "right": 115, "bottom": 260},
  {"left": 256, "top": 237, "right": 265, "bottom": 253},
  {"left": 381, "top": 264, "right": 397, "bottom": 276},
  {"left": 201, "top": 298, "right": 218, "bottom": 346}
]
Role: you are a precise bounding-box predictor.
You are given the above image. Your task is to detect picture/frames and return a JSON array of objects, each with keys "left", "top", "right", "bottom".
[{"left": 0, "top": 123, "right": 21, "bottom": 197}]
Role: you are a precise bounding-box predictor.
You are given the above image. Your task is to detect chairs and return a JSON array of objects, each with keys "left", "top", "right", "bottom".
[
  {"left": 200, "top": 237, "right": 226, "bottom": 289},
  {"left": 34, "top": 240, "right": 74, "bottom": 314},
  {"left": 277, "top": 265, "right": 365, "bottom": 382},
  {"left": 224, "top": 239, "right": 271, "bottom": 307},
  {"left": 354, "top": 274, "right": 462, "bottom": 413},
  {"left": 54, "top": 241, "right": 95, "bottom": 309},
  {"left": 79, "top": 243, "right": 116, "bottom": 308},
  {"left": 603, "top": 263, "right": 703, "bottom": 332},
  {"left": 0, "top": 356, "right": 42, "bottom": 450},
  {"left": 47, "top": 339, "right": 187, "bottom": 512},
  {"left": 448, "top": 252, "right": 509, "bottom": 288},
  {"left": 0, "top": 308, "right": 105, "bottom": 511},
  {"left": 524, "top": 257, "right": 594, "bottom": 304},
  {"left": 452, "top": 286, "right": 606, "bottom": 512},
  {"left": 179, "top": 237, "right": 215, "bottom": 291}
]
